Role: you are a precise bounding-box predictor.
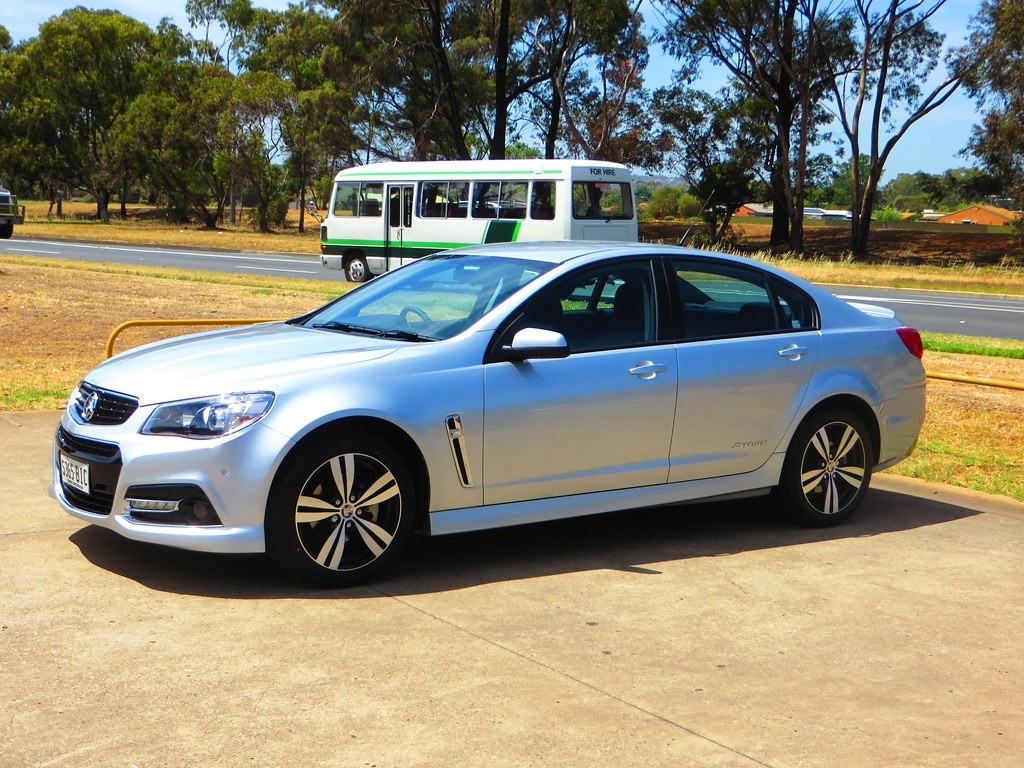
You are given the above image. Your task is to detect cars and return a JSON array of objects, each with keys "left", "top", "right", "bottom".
[{"left": 53, "top": 240, "right": 925, "bottom": 585}]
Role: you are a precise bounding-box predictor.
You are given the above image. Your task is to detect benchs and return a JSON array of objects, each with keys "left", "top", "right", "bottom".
[{"left": 683, "top": 300, "right": 783, "bottom": 334}]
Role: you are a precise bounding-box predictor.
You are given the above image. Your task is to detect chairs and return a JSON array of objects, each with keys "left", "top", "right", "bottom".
[
  {"left": 424, "top": 202, "right": 553, "bottom": 219},
  {"left": 604, "top": 283, "right": 645, "bottom": 346},
  {"left": 362, "top": 199, "right": 380, "bottom": 216},
  {"left": 528, "top": 297, "right": 566, "bottom": 333}
]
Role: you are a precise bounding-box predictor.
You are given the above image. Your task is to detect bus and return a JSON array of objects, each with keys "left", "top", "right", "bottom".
[{"left": 309, "top": 159, "right": 638, "bottom": 282}]
